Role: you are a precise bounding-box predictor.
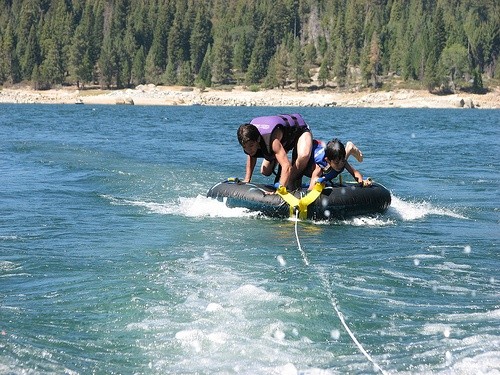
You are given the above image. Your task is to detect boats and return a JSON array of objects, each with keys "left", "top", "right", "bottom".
[{"left": 206, "top": 177, "right": 392, "bottom": 224}]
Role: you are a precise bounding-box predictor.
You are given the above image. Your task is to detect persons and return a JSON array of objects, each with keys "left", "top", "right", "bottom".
[
  {"left": 237, "top": 114, "right": 313, "bottom": 195},
  {"left": 289, "top": 140, "right": 368, "bottom": 192}
]
